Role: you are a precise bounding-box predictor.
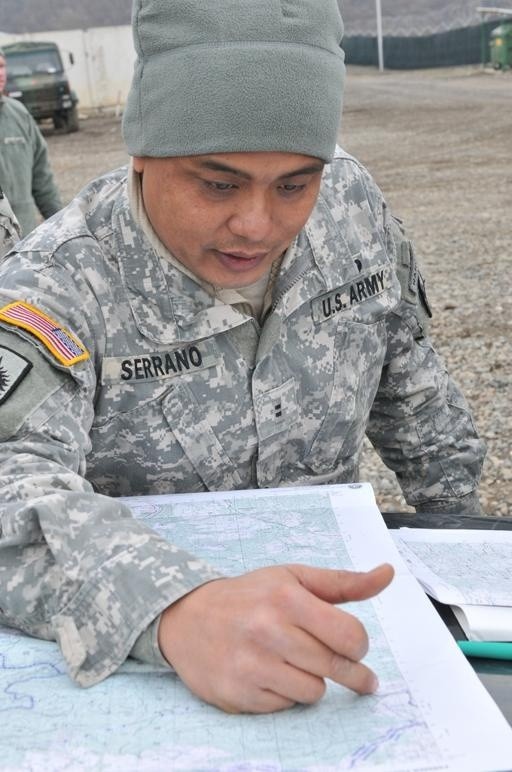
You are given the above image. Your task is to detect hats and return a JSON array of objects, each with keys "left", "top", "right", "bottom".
[{"left": 123, "top": 0, "right": 345, "bottom": 165}]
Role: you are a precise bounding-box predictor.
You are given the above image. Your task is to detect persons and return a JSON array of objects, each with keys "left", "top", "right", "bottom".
[
  {"left": 0, "top": 0, "right": 484, "bottom": 714},
  {"left": 0, "top": 52, "right": 65, "bottom": 258}
]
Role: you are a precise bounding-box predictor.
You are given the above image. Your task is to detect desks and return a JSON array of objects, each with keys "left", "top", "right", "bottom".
[{"left": 386, "top": 513, "right": 512, "bottom": 673}]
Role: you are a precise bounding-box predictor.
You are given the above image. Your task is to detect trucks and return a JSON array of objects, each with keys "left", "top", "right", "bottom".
[{"left": 0, "top": 39, "right": 79, "bottom": 137}]
